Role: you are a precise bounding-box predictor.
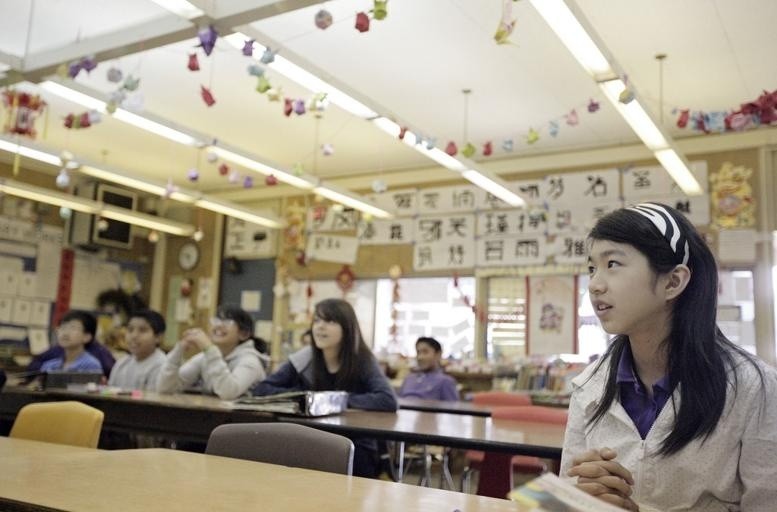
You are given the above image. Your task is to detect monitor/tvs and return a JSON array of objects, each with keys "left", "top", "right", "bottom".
[{"left": 64, "top": 180, "right": 138, "bottom": 250}]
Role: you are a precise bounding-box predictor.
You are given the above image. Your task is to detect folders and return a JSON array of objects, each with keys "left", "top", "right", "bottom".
[{"left": 231, "top": 390, "right": 351, "bottom": 416}]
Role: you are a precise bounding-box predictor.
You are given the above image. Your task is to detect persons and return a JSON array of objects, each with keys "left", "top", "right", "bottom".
[
  {"left": 372, "top": 334, "right": 462, "bottom": 478},
  {"left": 25, "top": 319, "right": 117, "bottom": 378},
  {"left": 299, "top": 327, "right": 315, "bottom": 350},
  {"left": 152, "top": 301, "right": 273, "bottom": 453},
  {"left": 95, "top": 288, "right": 135, "bottom": 355},
  {"left": 248, "top": 299, "right": 399, "bottom": 479},
  {"left": 556, "top": 200, "right": 777, "bottom": 512},
  {"left": 41, "top": 309, "right": 105, "bottom": 376},
  {"left": 98, "top": 309, "right": 167, "bottom": 452}
]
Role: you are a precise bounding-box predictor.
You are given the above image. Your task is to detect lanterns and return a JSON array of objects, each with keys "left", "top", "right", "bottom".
[{"left": 0, "top": 89, "right": 50, "bottom": 178}]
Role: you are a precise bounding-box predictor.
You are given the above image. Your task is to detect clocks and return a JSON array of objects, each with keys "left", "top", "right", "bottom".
[{"left": 176, "top": 241, "right": 200, "bottom": 272}]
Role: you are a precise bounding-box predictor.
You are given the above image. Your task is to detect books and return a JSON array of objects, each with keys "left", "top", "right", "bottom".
[
  {"left": 506, "top": 470, "right": 632, "bottom": 512},
  {"left": 378, "top": 352, "right": 589, "bottom": 405}
]
